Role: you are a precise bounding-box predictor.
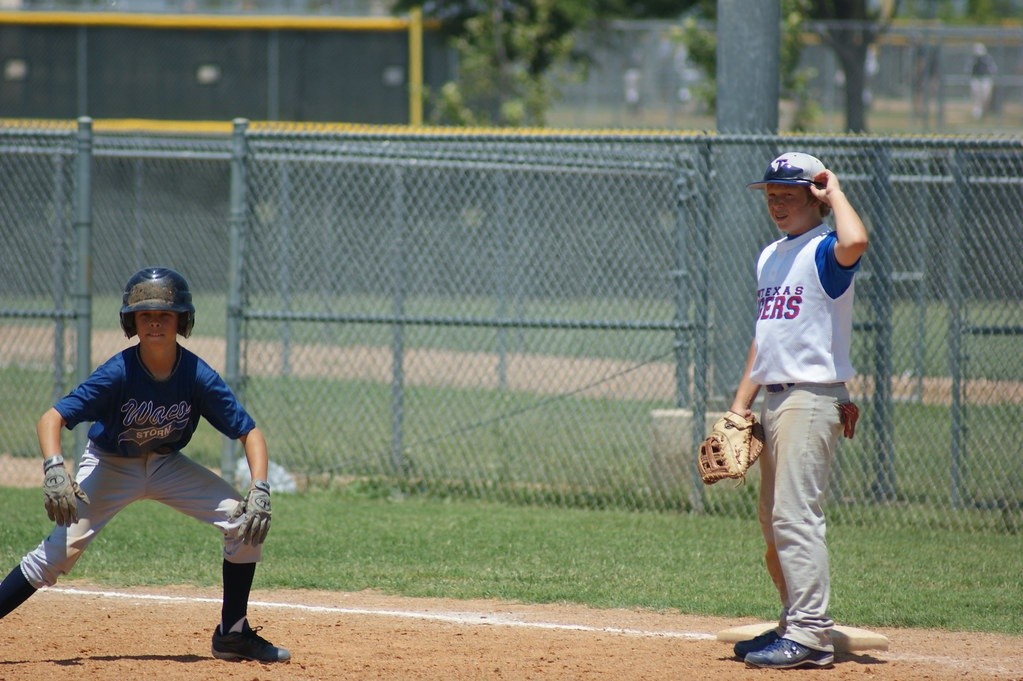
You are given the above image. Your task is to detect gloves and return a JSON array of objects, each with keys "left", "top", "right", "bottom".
[
  {"left": 841, "top": 402, "right": 859, "bottom": 439},
  {"left": 226, "top": 479, "right": 272, "bottom": 546},
  {"left": 41, "top": 454, "right": 90, "bottom": 529}
]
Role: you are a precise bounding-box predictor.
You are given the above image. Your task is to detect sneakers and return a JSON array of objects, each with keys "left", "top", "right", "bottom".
[
  {"left": 743, "top": 637, "right": 834, "bottom": 670},
  {"left": 733, "top": 631, "right": 783, "bottom": 658},
  {"left": 210, "top": 619, "right": 291, "bottom": 664}
]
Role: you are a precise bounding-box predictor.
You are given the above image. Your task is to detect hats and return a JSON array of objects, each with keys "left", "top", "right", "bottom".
[{"left": 746, "top": 151, "right": 827, "bottom": 188}]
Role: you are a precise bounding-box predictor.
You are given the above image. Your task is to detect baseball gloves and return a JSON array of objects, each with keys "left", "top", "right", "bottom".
[{"left": 697, "top": 411, "right": 767, "bottom": 485}]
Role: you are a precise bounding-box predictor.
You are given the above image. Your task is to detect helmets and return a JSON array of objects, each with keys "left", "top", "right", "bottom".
[{"left": 119, "top": 267, "right": 195, "bottom": 340}]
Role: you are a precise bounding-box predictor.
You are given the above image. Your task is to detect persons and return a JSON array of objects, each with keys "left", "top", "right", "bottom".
[
  {"left": 697, "top": 151, "right": 871, "bottom": 670},
  {"left": 0, "top": 266, "right": 291, "bottom": 667}
]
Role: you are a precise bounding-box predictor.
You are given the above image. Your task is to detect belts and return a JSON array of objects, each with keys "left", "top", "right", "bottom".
[{"left": 765, "top": 382, "right": 795, "bottom": 393}]
